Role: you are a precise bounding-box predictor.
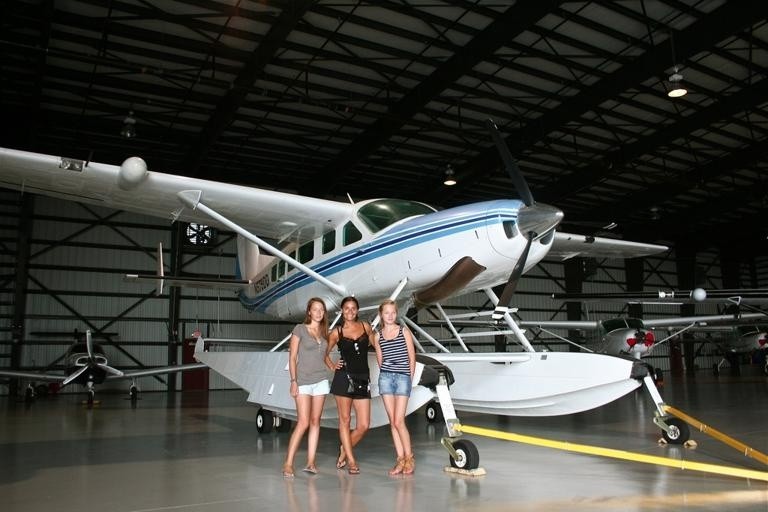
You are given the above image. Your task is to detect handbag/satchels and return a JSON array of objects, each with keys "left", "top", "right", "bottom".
[{"left": 347, "top": 375, "right": 370, "bottom": 395}]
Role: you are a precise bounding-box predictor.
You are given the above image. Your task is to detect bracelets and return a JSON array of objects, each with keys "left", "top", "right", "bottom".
[{"left": 290, "top": 379, "right": 295, "bottom": 383}]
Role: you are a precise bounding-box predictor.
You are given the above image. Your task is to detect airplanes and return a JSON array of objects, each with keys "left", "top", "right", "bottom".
[
  {"left": 419, "top": 310, "right": 767, "bottom": 391},
  {"left": 652, "top": 322, "right": 767, "bottom": 381},
  {"left": 0, "top": 326, "right": 213, "bottom": 409},
  {"left": 0, "top": 116, "right": 690, "bottom": 472}
]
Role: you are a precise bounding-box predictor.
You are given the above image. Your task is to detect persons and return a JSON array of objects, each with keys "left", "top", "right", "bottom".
[
  {"left": 282, "top": 297, "right": 338, "bottom": 477},
  {"left": 373, "top": 300, "right": 416, "bottom": 474},
  {"left": 324, "top": 297, "right": 383, "bottom": 474}
]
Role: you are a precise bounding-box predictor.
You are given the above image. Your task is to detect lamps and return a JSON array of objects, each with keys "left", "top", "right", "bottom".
[
  {"left": 440, "top": 161, "right": 460, "bottom": 189},
  {"left": 663, "top": 63, "right": 690, "bottom": 103},
  {"left": 115, "top": 107, "right": 143, "bottom": 144}
]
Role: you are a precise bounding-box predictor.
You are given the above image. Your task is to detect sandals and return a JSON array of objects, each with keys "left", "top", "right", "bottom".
[{"left": 283, "top": 445, "right": 414, "bottom": 477}]
